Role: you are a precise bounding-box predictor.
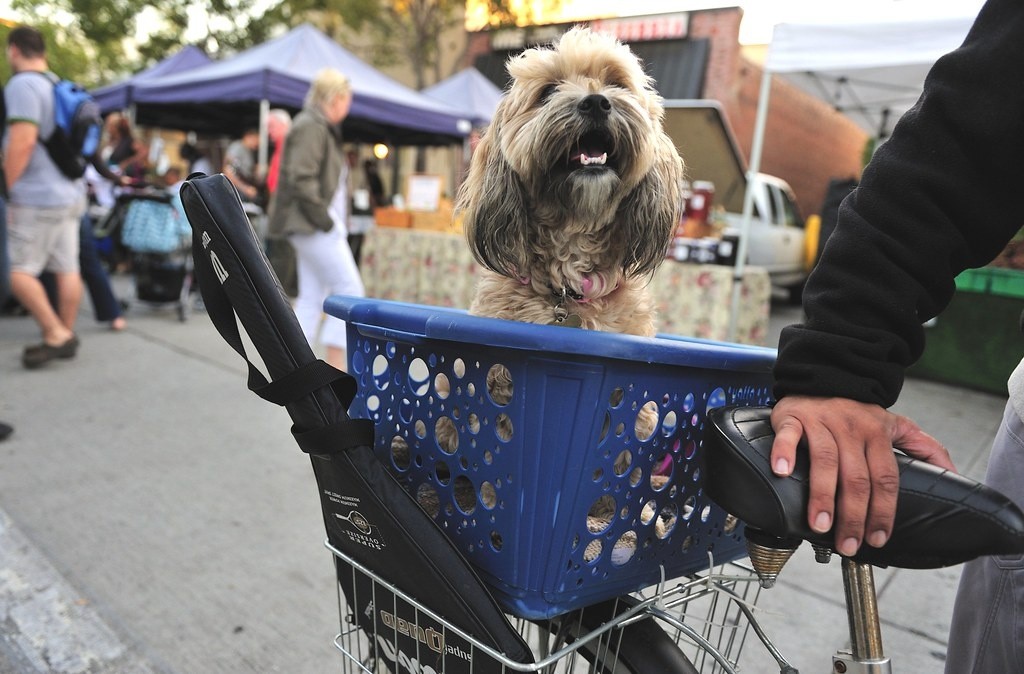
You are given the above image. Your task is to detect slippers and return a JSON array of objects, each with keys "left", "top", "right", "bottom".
[{"left": 21, "top": 336, "right": 79, "bottom": 366}]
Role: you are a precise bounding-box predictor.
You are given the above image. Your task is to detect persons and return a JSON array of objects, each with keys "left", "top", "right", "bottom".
[
  {"left": 78, "top": 112, "right": 182, "bottom": 330},
  {"left": 0, "top": 26, "right": 82, "bottom": 368},
  {"left": 178, "top": 143, "right": 210, "bottom": 178},
  {"left": 271, "top": 71, "right": 366, "bottom": 367},
  {"left": 356, "top": 160, "right": 385, "bottom": 266},
  {"left": 460, "top": 127, "right": 490, "bottom": 210},
  {"left": 267, "top": 109, "right": 293, "bottom": 208},
  {"left": 346, "top": 150, "right": 359, "bottom": 209},
  {"left": 771, "top": 0, "right": 1024, "bottom": 673}
]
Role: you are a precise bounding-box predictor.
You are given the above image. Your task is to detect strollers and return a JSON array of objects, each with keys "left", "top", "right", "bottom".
[{"left": 107, "top": 175, "right": 208, "bottom": 322}]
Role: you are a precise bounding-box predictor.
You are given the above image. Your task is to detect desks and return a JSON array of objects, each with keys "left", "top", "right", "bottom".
[{"left": 358, "top": 224, "right": 774, "bottom": 347}]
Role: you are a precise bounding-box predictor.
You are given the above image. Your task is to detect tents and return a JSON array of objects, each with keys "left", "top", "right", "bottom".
[
  {"left": 730, "top": 0, "right": 987, "bottom": 336},
  {"left": 90, "top": 25, "right": 507, "bottom": 248}
]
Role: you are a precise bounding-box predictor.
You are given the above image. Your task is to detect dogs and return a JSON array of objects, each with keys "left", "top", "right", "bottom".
[{"left": 415, "top": 23, "right": 690, "bottom": 540}]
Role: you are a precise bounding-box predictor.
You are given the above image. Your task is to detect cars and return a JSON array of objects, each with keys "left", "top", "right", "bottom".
[{"left": 715, "top": 171, "right": 818, "bottom": 305}]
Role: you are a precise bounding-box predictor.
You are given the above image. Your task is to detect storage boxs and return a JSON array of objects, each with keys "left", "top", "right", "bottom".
[{"left": 322, "top": 294, "right": 778, "bottom": 622}]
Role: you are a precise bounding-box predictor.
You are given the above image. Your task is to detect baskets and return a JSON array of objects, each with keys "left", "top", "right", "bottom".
[{"left": 323, "top": 292, "right": 778, "bottom": 618}]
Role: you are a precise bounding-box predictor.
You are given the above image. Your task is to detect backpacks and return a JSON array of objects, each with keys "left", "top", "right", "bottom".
[{"left": 17, "top": 70, "right": 102, "bottom": 179}]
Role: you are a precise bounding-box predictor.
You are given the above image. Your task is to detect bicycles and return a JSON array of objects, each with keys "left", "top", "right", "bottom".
[{"left": 320, "top": 402, "right": 1024, "bottom": 672}]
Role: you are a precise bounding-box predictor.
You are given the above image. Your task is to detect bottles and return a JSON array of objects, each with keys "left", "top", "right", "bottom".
[{"left": 666, "top": 180, "right": 741, "bottom": 266}]
